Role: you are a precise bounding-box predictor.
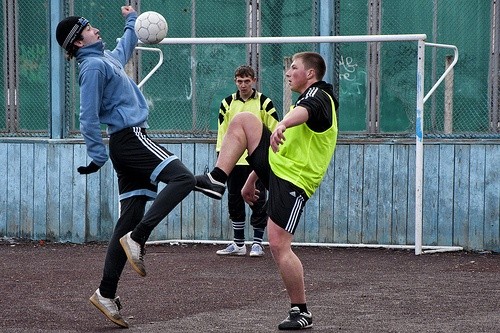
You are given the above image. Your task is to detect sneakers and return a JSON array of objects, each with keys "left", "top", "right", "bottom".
[
  {"left": 249, "top": 244, "right": 264, "bottom": 257},
  {"left": 119, "top": 231, "right": 148, "bottom": 277},
  {"left": 89, "top": 287, "right": 129, "bottom": 328},
  {"left": 216, "top": 242, "right": 247, "bottom": 256},
  {"left": 193, "top": 173, "right": 226, "bottom": 200},
  {"left": 278, "top": 306, "right": 313, "bottom": 329}
]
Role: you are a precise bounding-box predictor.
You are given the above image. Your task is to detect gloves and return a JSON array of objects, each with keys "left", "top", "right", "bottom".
[{"left": 77, "top": 161, "right": 101, "bottom": 175}]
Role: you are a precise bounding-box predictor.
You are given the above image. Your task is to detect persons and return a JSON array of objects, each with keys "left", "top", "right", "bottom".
[
  {"left": 55, "top": 5, "right": 197, "bottom": 328},
  {"left": 192, "top": 51, "right": 339, "bottom": 330},
  {"left": 216, "top": 66, "right": 280, "bottom": 257}
]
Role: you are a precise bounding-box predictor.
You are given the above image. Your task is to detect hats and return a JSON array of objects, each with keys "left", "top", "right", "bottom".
[{"left": 56, "top": 16, "right": 90, "bottom": 52}]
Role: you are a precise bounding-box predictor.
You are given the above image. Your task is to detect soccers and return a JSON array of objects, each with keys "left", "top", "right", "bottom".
[{"left": 134, "top": 11, "right": 168, "bottom": 45}]
